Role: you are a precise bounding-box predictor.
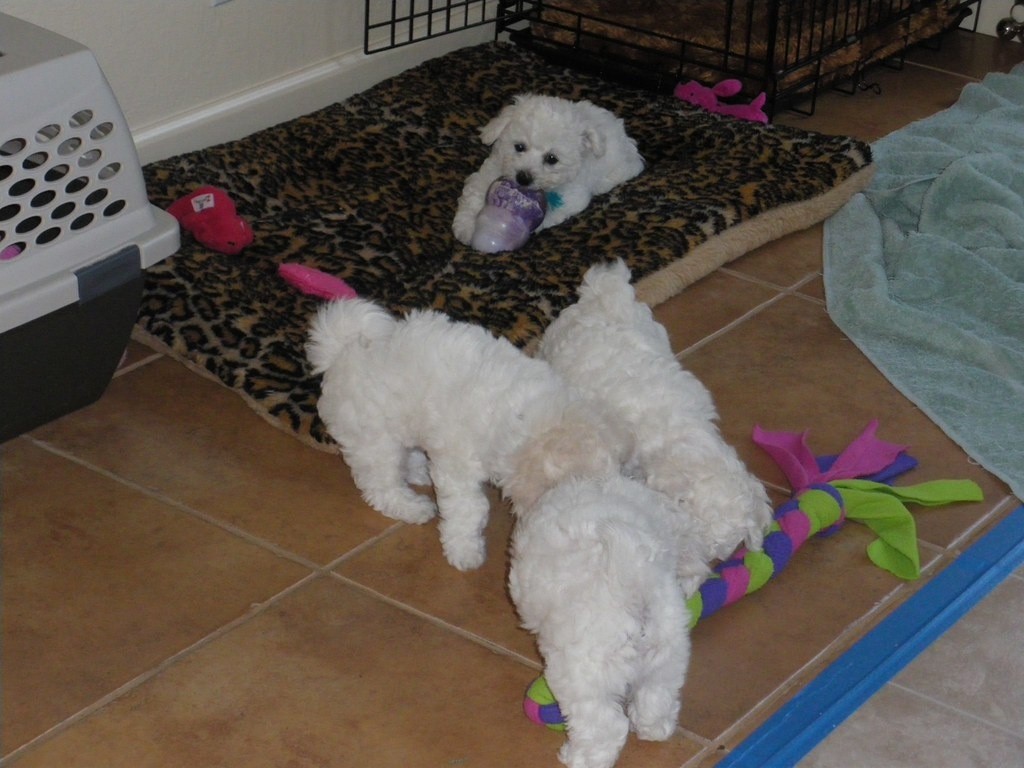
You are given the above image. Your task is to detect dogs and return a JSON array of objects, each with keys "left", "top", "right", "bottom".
[
  {"left": 452, "top": 91, "right": 646, "bottom": 247},
  {"left": 303, "top": 257, "right": 775, "bottom": 768}
]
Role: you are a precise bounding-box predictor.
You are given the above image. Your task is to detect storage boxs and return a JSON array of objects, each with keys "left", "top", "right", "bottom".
[{"left": 0, "top": 11, "right": 182, "bottom": 441}]
